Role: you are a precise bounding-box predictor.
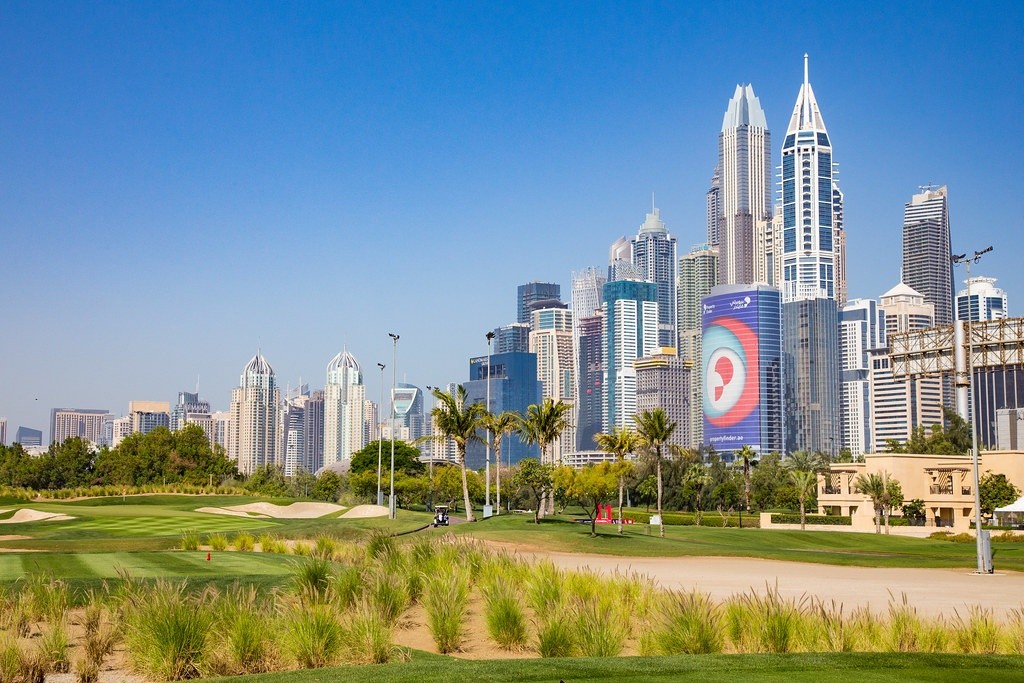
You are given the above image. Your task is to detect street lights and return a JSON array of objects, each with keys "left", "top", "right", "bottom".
[
  {"left": 485, "top": 331, "right": 497, "bottom": 506},
  {"left": 388, "top": 333, "right": 400, "bottom": 519},
  {"left": 377, "top": 363, "right": 385, "bottom": 506},
  {"left": 947, "top": 246, "right": 992, "bottom": 575}
]
